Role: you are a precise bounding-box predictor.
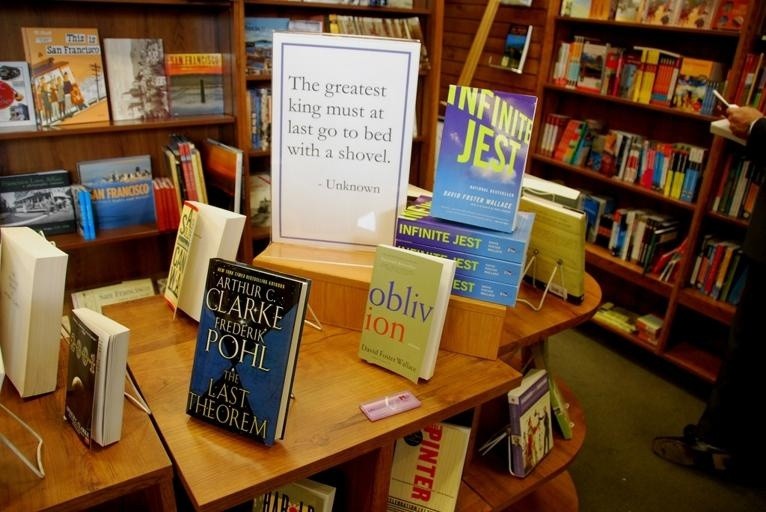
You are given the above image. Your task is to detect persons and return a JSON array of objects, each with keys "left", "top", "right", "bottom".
[{"left": 652, "top": 107, "right": 766, "bottom": 480}]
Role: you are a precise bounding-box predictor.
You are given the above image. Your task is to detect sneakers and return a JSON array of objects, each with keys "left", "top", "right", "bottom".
[{"left": 651, "top": 434, "right": 758, "bottom": 490}]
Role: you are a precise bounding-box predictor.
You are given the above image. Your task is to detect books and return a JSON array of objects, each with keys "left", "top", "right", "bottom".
[
  {"left": 480, "top": 357, "right": 571, "bottom": 478},
  {"left": 0, "top": 0, "right": 539, "bottom": 306},
  {"left": 253, "top": 479, "right": 336, "bottom": 512},
  {"left": 358, "top": 245, "right": 457, "bottom": 385},
  {"left": 520, "top": 0, "right": 766, "bottom": 347},
  {"left": 388, "top": 423, "right": 471, "bottom": 512},
  {"left": 64, "top": 308, "right": 130, "bottom": 449},
  {"left": 1, "top": 227, "right": 68, "bottom": 397},
  {"left": 186, "top": 257, "right": 309, "bottom": 446}
]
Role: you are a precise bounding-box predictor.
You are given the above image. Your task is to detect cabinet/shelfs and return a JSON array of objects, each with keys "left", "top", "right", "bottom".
[{"left": 0, "top": 0, "right": 765, "bottom": 511}]
